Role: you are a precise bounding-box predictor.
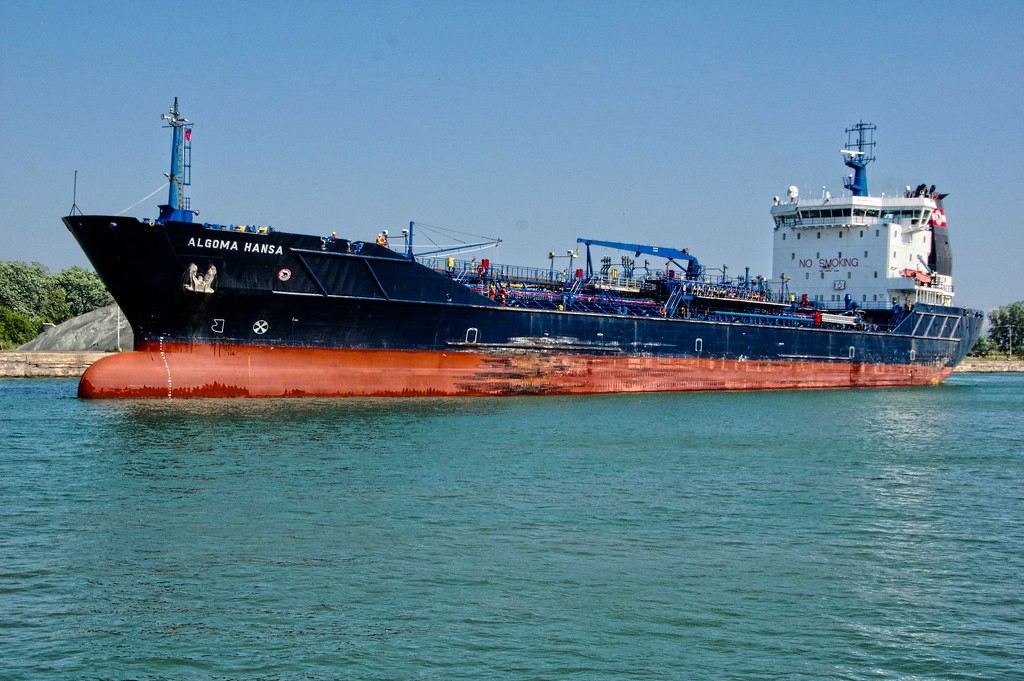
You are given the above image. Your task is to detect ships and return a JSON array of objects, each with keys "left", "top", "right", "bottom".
[{"left": 61, "top": 96, "right": 985, "bottom": 400}]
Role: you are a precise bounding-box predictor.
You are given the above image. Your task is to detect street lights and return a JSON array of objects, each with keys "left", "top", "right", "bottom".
[{"left": 1006, "top": 324, "right": 1011, "bottom": 358}]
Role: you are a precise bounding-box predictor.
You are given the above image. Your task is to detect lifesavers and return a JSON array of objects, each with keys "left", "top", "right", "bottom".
[{"left": 659, "top": 306, "right": 664, "bottom": 314}]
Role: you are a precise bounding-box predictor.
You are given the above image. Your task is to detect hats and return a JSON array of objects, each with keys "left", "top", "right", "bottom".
[{"left": 379, "top": 234, "right": 382, "bottom": 237}]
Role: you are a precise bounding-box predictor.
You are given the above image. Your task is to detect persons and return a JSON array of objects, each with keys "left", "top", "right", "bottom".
[
  {"left": 501, "top": 285, "right": 509, "bottom": 306},
  {"left": 376, "top": 235, "right": 387, "bottom": 247},
  {"left": 814, "top": 310, "right": 821, "bottom": 327},
  {"left": 471, "top": 256, "right": 477, "bottom": 272},
  {"left": 908, "top": 300, "right": 912, "bottom": 311},
  {"left": 489, "top": 285, "right": 496, "bottom": 299},
  {"left": 561, "top": 270, "right": 566, "bottom": 282},
  {"left": 333, "top": 232, "right": 336, "bottom": 239},
  {"left": 692, "top": 285, "right": 760, "bottom": 301}
]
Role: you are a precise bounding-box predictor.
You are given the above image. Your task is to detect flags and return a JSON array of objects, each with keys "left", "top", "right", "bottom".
[{"left": 184, "top": 129, "right": 192, "bottom": 141}]
